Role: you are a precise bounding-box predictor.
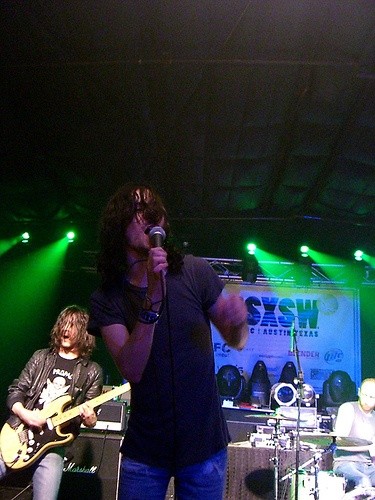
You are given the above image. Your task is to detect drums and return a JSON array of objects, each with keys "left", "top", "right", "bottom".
[
  {"left": 297, "top": 384, "right": 315, "bottom": 405},
  {"left": 287, "top": 470, "right": 347, "bottom": 500},
  {"left": 271, "top": 382, "right": 297, "bottom": 405}
]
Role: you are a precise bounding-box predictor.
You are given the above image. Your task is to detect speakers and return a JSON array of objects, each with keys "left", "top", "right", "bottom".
[{"left": 57, "top": 430, "right": 125, "bottom": 500}]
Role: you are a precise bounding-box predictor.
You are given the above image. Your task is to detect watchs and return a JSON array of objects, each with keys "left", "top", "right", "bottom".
[{"left": 138, "top": 308, "right": 161, "bottom": 327}]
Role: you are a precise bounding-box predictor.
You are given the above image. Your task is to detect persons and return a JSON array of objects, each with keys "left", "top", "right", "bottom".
[
  {"left": 4, "top": 303, "right": 105, "bottom": 500},
  {"left": 331, "top": 378, "right": 375, "bottom": 500},
  {"left": 79, "top": 182, "right": 252, "bottom": 500}
]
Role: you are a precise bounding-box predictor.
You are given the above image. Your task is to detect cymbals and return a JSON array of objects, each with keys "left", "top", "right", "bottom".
[{"left": 299, "top": 435, "right": 373, "bottom": 447}]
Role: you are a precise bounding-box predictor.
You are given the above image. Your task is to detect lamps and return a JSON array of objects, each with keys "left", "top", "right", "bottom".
[{"left": 267, "top": 382, "right": 298, "bottom": 408}]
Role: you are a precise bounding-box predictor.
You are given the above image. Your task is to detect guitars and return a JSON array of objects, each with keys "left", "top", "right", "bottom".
[{"left": 0, "top": 384, "right": 129, "bottom": 472}]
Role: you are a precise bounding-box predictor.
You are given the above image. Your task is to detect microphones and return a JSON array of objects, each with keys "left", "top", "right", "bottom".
[
  {"left": 148, "top": 226, "right": 167, "bottom": 269},
  {"left": 290, "top": 321, "right": 295, "bottom": 352}
]
